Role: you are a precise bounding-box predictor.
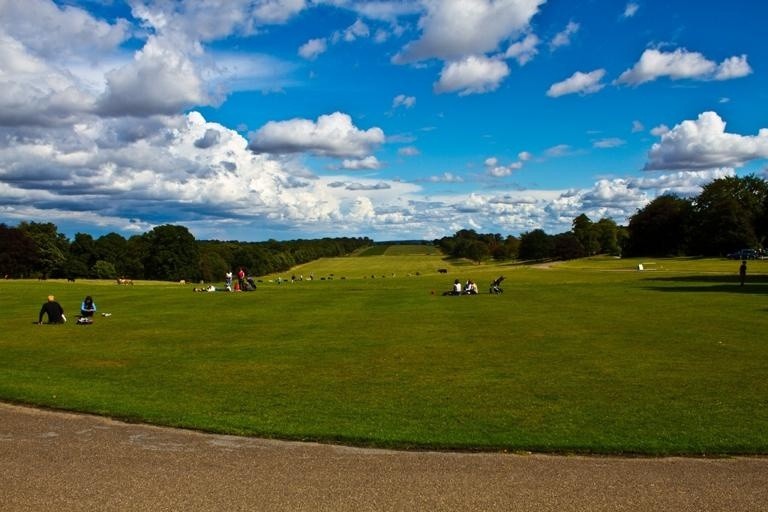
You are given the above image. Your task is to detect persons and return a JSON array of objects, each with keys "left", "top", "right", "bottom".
[
  {"left": 193, "top": 277, "right": 256, "bottom": 293},
  {"left": 37, "top": 295, "right": 65, "bottom": 325},
  {"left": 739, "top": 261, "right": 747, "bottom": 286},
  {"left": 236, "top": 268, "right": 244, "bottom": 290},
  {"left": 80, "top": 295, "right": 97, "bottom": 317},
  {"left": 225, "top": 268, "right": 233, "bottom": 284},
  {"left": 463, "top": 278, "right": 479, "bottom": 296},
  {"left": 493, "top": 275, "right": 505, "bottom": 296},
  {"left": 441, "top": 278, "right": 461, "bottom": 297}
]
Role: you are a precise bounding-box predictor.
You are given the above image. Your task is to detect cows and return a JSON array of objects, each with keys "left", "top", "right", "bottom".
[{"left": 437, "top": 269, "right": 448, "bottom": 275}]
